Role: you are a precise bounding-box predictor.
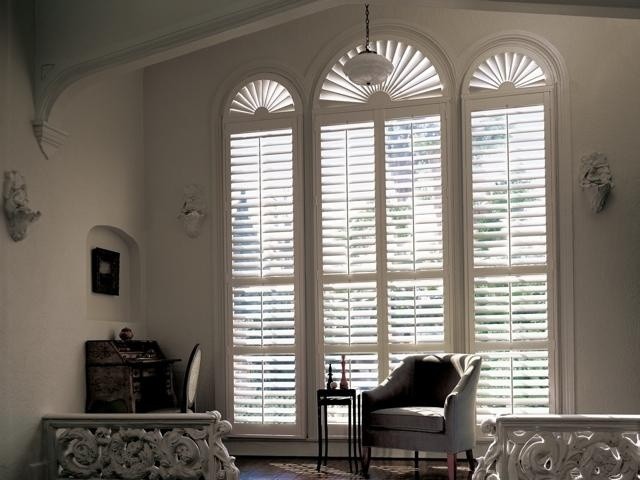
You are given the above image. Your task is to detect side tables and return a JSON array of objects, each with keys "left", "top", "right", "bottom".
[{"left": 315, "top": 386, "right": 360, "bottom": 478}]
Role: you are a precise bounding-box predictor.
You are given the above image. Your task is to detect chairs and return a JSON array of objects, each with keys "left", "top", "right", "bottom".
[
  {"left": 353, "top": 352, "right": 481, "bottom": 480},
  {"left": 141, "top": 341, "right": 203, "bottom": 416}
]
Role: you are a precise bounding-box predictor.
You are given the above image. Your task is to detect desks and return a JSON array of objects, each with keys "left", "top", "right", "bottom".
[{"left": 78, "top": 338, "right": 183, "bottom": 413}]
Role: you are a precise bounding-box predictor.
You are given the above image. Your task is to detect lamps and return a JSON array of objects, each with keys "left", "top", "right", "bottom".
[{"left": 343, "top": 1, "right": 395, "bottom": 86}]
